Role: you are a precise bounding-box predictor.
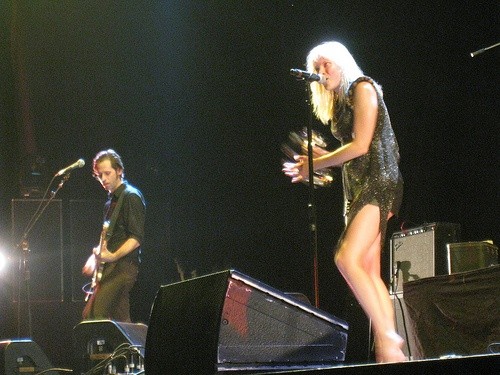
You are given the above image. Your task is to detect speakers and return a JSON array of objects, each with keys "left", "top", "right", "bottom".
[
  {"left": 0, "top": 340, "right": 60, "bottom": 375},
  {"left": 73, "top": 320, "right": 148, "bottom": 374},
  {"left": 389, "top": 222, "right": 461, "bottom": 294},
  {"left": 144, "top": 269, "right": 348, "bottom": 375}
]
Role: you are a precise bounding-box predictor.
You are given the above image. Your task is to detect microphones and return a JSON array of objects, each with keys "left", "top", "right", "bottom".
[
  {"left": 58, "top": 159, "right": 85, "bottom": 176},
  {"left": 396, "top": 261, "right": 401, "bottom": 278},
  {"left": 290, "top": 69, "right": 325, "bottom": 84}
]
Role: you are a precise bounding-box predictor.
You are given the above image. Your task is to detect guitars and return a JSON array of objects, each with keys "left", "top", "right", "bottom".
[{"left": 84, "top": 219, "right": 112, "bottom": 290}]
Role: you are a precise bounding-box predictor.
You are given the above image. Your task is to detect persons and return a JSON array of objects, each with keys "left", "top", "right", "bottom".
[
  {"left": 81, "top": 148, "right": 146, "bottom": 321},
  {"left": 283, "top": 41, "right": 407, "bottom": 364}
]
